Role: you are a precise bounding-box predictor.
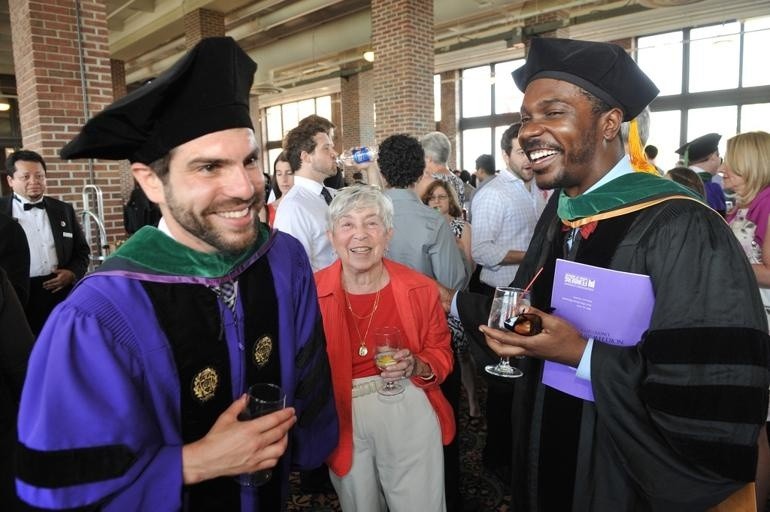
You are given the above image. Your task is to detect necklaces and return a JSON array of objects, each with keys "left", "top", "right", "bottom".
[{"left": 342, "top": 268, "right": 384, "bottom": 358}]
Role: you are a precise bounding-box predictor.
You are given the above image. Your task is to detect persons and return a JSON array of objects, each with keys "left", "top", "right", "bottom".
[
  {"left": 671, "top": 133, "right": 725, "bottom": 216},
  {"left": 14, "top": 35, "right": 341, "bottom": 511},
  {"left": 376, "top": 134, "right": 468, "bottom": 291},
  {"left": 0, "top": 149, "right": 91, "bottom": 337},
  {"left": 436, "top": 37, "right": 769, "bottom": 512},
  {"left": 266, "top": 149, "right": 294, "bottom": 231},
  {"left": 313, "top": 184, "right": 456, "bottom": 512},
  {"left": 642, "top": 145, "right": 665, "bottom": 178},
  {"left": 297, "top": 114, "right": 345, "bottom": 192},
  {"left": 717, "top": 131, "right": 770, "bottom": 321},
  {"left": 421, "top": 179, "right": 471, "bottom": 266},
  {"left": 348, "top": 169, "right": 369, "bottom": 189},
  {"left": 268, "top": 122, "right": 385, "bottom": 277},
  {"left": 473, "top": 154, "right": 498, "bottom": 194},
  {"left": 466, "top": 154, "right": 487, "bottom": 198},
  {"left": 413, "top": 130, "right": 466, "bottom": 208},
  {"left": 470, "top": 123, "right": 555, "bottom": 291}
]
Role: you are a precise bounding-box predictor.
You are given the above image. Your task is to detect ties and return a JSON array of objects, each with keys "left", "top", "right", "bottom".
[{"left": 321, "top": 184, "right": 333, "bottom": 206}]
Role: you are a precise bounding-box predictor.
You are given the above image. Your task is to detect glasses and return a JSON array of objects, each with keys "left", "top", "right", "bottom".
[
  {"left": 504, "top": 306, "right": 556, "bottom": 336},
  {"left": 430, "top": 192, "right": 450, "bottom": 200}
]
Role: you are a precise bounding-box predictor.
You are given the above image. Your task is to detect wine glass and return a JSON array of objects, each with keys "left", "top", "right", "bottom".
[
  {"left": 232, "top": 383, "right": 286, "bottom": 488},
  {"left": 485, "top": 287, "right": 533, "bottom": 378},
  {"left": 373, "top": 326, "right": 405, "bottom": 396}
]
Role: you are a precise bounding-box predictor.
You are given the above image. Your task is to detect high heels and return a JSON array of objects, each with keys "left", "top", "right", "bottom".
[{"left": 469, "top": 402, "right": 483, "bottom": 428}]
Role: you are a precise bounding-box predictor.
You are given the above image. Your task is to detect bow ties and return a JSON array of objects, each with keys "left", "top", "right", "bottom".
[{"left": 22, "top": 202, "right": 44, "bottom": 211}]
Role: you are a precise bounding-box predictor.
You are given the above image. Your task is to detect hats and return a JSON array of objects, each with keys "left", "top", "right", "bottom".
[
  {"left": 57, "top": 35, "right": 257, "bottom": 169},
  {"left": 675, "top": 133, "right": 722, "bottom": 166},
  {"left": 509, "top": 36, "right": 660, "bottom": 124}
]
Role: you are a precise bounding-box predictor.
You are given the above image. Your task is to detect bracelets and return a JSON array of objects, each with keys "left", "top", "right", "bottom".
[{"left": 417, "top": 370, "right": 441, "bottom": 382}]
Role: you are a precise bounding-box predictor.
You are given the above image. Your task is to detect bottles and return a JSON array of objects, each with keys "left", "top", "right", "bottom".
[{"left": 336, "top": 145, "right": 381, "bottom": 167}]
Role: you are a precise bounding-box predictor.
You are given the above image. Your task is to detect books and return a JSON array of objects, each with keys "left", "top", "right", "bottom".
[{"left": 541, "top": 258, "right": 656, "bottom": 404}]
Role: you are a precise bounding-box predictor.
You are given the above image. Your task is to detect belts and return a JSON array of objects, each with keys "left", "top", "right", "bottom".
[{"left": 349, "top": 377, "right": 410, "bottom": 398}]
